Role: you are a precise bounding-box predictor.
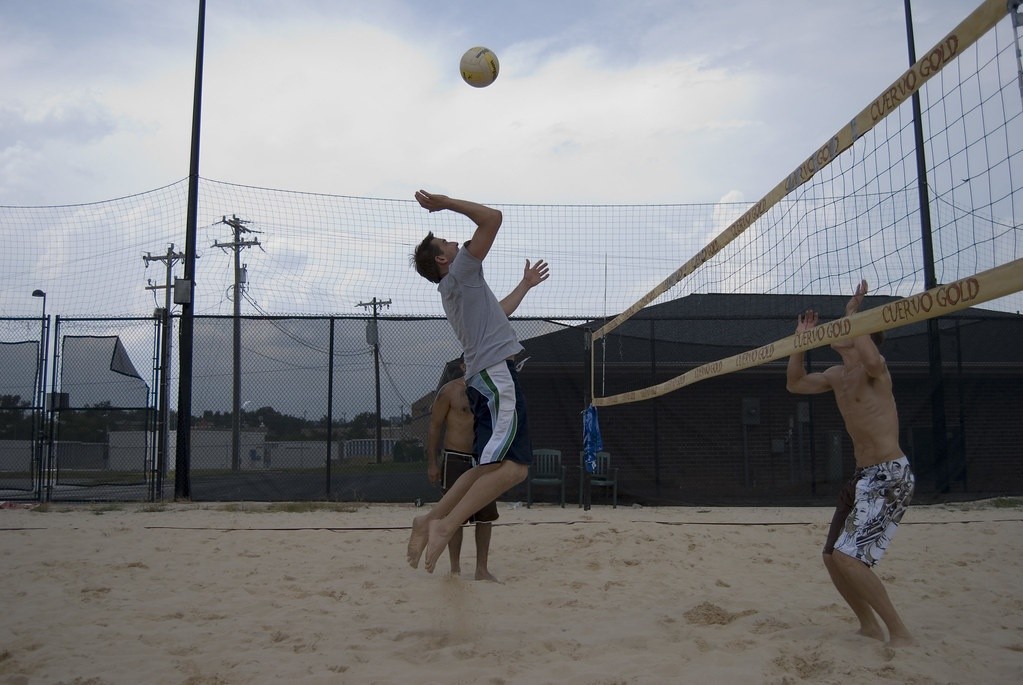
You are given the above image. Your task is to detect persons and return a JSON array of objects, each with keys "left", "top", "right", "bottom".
[
  {"left": 404, "top": 190, "right": 549, "bottom": 585},
  {"left": 786, "top": 279, "right": 916, "bottom": 648}
]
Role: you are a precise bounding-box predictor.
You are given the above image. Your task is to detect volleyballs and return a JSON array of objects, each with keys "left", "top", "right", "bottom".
[{"left": 458, "top": 46, "right": 501, "bottom": 89}]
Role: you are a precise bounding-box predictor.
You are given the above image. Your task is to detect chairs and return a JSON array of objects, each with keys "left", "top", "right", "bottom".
[
  {"left": 579, "top": 450, "right": 618, "bottom": 510},
  {"left": 526, "top": 449, "right": 567, "bottom": 508}
]
